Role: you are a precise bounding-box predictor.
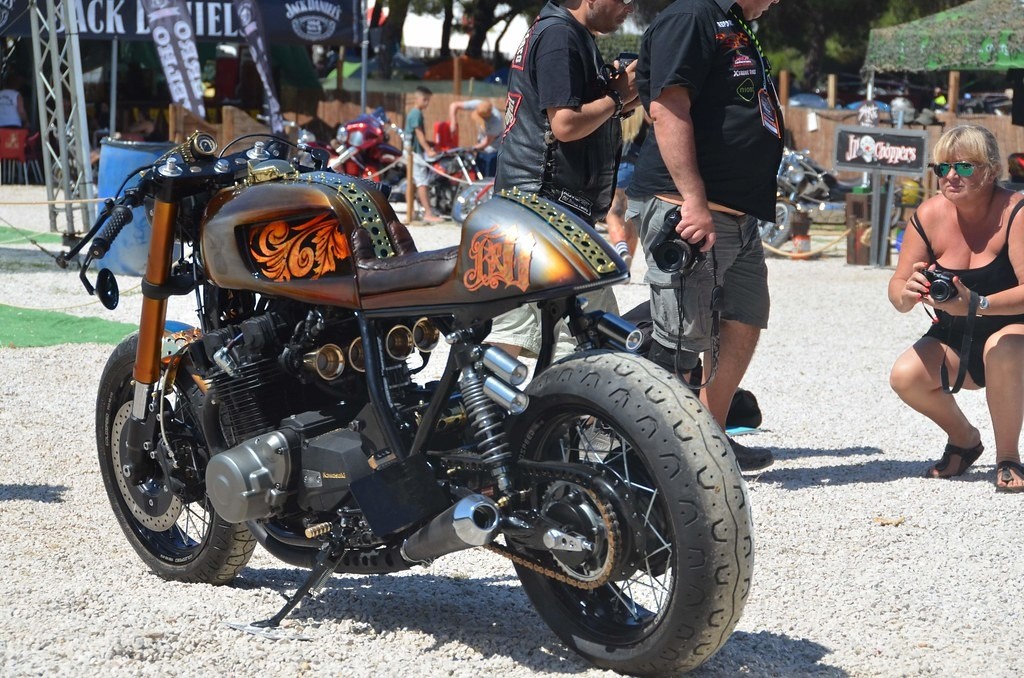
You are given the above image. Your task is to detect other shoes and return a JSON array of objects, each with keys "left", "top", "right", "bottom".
[
  {"left": 432, "top": 210, "right": 444, "bottom": 216},
  {"left": 725, "top": 434, "right": 774, "bottom": 476},
  {"left": 618, "top": 251, "right": 632, "bottom": 270},
  {"left": 616, "top": 272, "right": 632, "bottom": 284},
  {"left": 422, "top": 214, "right": 445, "bottom": 225}
]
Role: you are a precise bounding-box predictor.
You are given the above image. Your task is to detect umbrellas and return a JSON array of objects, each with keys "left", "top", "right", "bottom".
[
  {"left": 350, "top": 53, "right": 511, "bottom": 84},
  {"left": 789, "top": 91, "right": 890, "bottom": 113}
]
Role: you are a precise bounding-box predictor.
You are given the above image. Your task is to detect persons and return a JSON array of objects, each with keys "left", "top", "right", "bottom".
[
  {"left": 0, "top": 79, "right": 154, "bottom": 170},
  {"left": 624, "top": 0, "right": 784, "bottom": 471},
  {"left": 888, "top": 123, "right": 1024, "bottom": 493},
  {"left": 404, "top": 87, "right": 445, "bottom": 226},
  {"left": 480, "top": 0, "right": 641, "bottom": 433},
  {"left": 449, "top": 98, "right": 503, "bottom": 177},
  {"left": 606, "top": 54, "right": 638, "bottom": 284}
]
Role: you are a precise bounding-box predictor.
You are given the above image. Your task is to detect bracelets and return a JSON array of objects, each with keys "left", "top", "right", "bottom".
[{"left": 608, "top": 90, "right": 622, "bottom": 118}]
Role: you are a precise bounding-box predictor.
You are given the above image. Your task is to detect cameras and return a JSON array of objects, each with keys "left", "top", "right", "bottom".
[
  {"left": 917, "top": 269, "right": 961, "bottom": 303},
  {"left": 648, "top": 211, "right": 708, "bottom": 273},
  {"left": 609, "top": 52, "right": 639, "bottom": 122}
]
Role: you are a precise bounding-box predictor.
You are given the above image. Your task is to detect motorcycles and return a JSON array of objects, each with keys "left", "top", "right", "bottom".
[
  {"left": 254, "top": 113, "right": 343, "bottom": 169},
  {"left": 325, "top": 103, "right": 484, "bottom": 223},
  {"left": 49, "top": 130, "right": 760, "bottom": 678},
  {"left": 450, "top": 173, "right": 496, "bottom": 225},
  {"left": 756, "top": 144, "right": 942, "bottom": 258}
]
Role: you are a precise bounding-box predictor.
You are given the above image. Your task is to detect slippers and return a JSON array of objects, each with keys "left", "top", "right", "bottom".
[
  {"left": 921, "top": 440, "right": 985, "bottom": 479},
  {"left": 994, "top": 460, "right": 1024, "bottom": 494}
]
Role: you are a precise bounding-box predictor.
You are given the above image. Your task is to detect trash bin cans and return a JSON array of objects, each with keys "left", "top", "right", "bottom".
[{"left": 93, "top": 137, "right": 181, "bottom": 278}]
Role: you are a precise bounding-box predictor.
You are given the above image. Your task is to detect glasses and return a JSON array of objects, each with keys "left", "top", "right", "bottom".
[
  {"left": 622, "top": 0, "right": 631, "bottom": 4},
  {"left": 933, "top": 161, "right": 986, "bottom": 178}
]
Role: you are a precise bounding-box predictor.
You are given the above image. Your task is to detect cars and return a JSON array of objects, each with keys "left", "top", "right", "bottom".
[
  {"left": 314, "top": 44, "right": 511, "bottom": 87},
  {"left": 787, "top": 81, "right": 1013, "bottom": 126}
]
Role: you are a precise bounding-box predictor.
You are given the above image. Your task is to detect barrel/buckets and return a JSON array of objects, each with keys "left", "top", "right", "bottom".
[
  {"left": 791, "top": 234, "right": 812, "bottom": 261},
  {"left": 95, "top": 137, "right": 182, "bottom": 277}
]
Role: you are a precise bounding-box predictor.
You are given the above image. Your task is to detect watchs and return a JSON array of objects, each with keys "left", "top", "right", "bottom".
[{"left": 977, "top": 296, "right": 989, "bottom": 315}]
